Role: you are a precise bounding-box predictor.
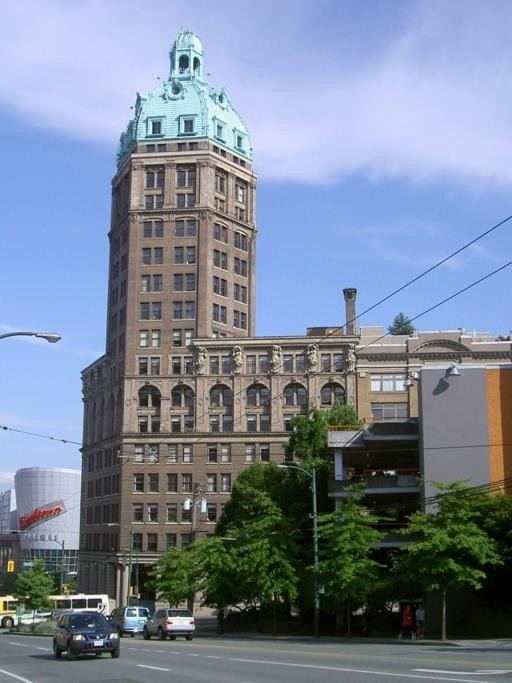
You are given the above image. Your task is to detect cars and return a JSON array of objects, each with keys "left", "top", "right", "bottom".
[{"left": 53, "top": 606, "right": 196, "bottom": 661}]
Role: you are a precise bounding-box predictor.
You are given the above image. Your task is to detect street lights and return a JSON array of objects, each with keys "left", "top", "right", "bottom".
[
  {"left": 0, "top": 331, "right": 64, "bottom": 343},
  {"left": 182, "top": 494, "right": 207, "bottom": 611},
  {"left": 275, "top": 464, "right": 321, "bottom": 636},
  {"left": 48, "top": 538, "right": 66, "bottom": 596},
  {"left": 106, "top": 524, "right": 133, "bottom": 605}
]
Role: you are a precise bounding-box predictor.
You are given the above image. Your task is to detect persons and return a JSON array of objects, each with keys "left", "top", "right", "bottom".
[
  {"left": 308, "top": 345, "right": 318, "bottom": 372},
  {"left": 271, "top": 345, "right": 281, "bottom": 373},
  {"left": 415, "top": 604, "right": 426, "bottom": 638},
  {"left": 196, "top": 347, "right": 207, "bottom": 375},
  {"left": 398, "top": 605, "right": 418, "bottom": 641},
  {"left": 232, "top": 345, "right": 243, "bottom": 372},
  {"left": 227, "top": 609, "right": 235, "bottom": 634},
  {"left": 217, "top": 609, "right": 224, "bottom": 635},
  {"left": 346, "top": 344, "right": 356, "bottom": 371}
]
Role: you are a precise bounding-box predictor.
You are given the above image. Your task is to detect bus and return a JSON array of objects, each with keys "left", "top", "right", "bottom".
[{"left": 0, "top": 594, "right": 110, "bottom": 628}]
